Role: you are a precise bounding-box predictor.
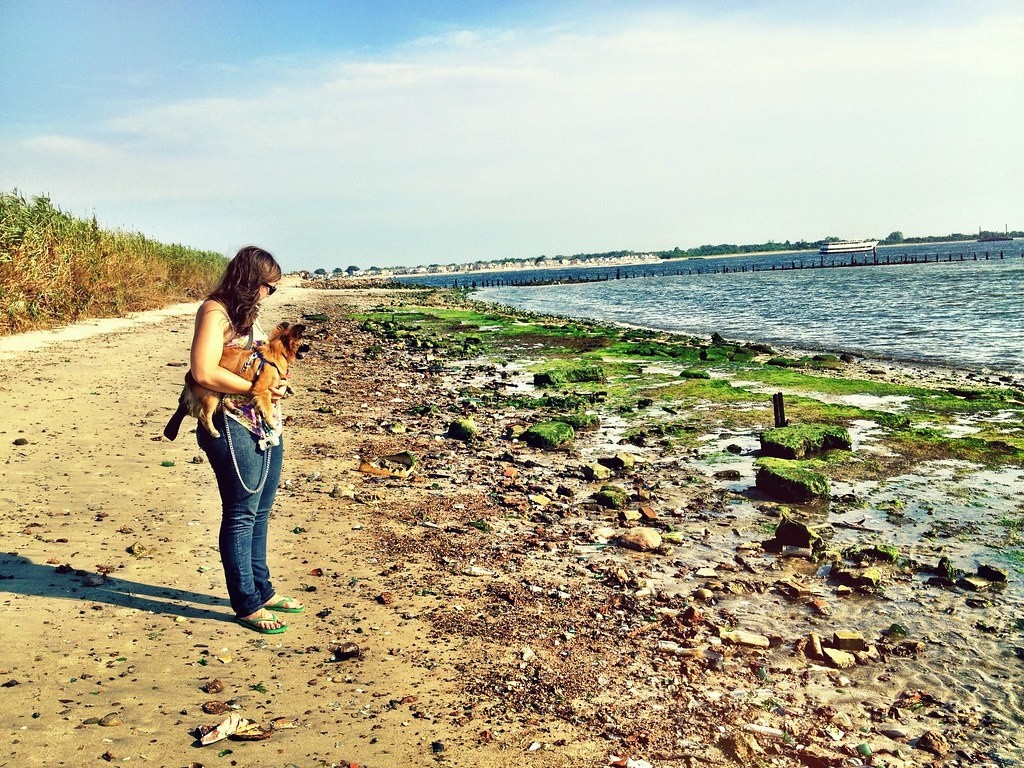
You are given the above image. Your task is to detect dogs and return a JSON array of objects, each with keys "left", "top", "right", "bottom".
[{"left": 179, "top": 321, "right": 312, "bottom": 439}]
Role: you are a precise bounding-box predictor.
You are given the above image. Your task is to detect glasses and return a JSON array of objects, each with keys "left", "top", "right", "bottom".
[{"left": 261, "top": 282, "right": 276, "bottom": 295}]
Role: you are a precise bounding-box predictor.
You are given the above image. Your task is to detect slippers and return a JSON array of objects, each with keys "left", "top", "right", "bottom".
[
  {"left": 264, "top": 595, "right": 304, "bottom": 613},
  {"left": 236, "top": 612, "right": 288, "bottom": 634}
]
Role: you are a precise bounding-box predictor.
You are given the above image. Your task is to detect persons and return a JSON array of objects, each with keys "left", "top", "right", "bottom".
[{"left": 191, "top": 246, "right": 305, "bottom": 634}]
